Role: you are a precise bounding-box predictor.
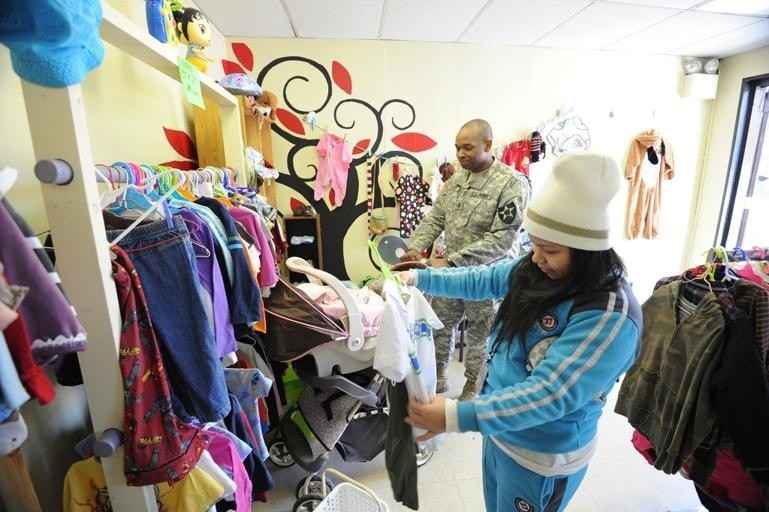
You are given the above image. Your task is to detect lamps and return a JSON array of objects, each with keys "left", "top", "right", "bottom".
[{"left": 680, "top": 56, "right": 720, "bottom": 100}]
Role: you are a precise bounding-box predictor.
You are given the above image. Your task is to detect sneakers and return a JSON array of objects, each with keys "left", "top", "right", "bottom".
[{"left": 290, "top": 204, "right": 314, "bottom": 245}]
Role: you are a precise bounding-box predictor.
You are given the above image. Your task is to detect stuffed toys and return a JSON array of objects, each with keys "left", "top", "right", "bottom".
[{"left": 251, "top": 87, "right": 283, "bottom": 123}]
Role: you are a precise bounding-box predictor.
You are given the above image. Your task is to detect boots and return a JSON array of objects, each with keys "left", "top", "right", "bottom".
[
  {"left": 436, "top": 368, "right": 449, "bottom": 393},
  {"left": 458, "top": 378, "right": 477, "bottom": 400}
]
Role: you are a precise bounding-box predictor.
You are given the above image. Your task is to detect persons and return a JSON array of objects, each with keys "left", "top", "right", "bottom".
[
  {"left": 401, "top": 156, "right": 643, "bottom": 512},
  {"left": 168, "top": 0, "right": 219, "bottom": 77},
  {"left": 404, "top": 116, "right": 531, "bottom": 403}
]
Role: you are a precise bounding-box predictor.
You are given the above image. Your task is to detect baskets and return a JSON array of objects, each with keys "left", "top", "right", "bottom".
[{"left": 313, "top": 467, "right": 390, "bottom": 512}]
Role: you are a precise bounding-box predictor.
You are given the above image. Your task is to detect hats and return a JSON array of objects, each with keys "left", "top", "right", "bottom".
[
  {"left": 522, "top": 152, "right": 621, "bottom": 252},
  {"left": 1, "top": 1, "right": 101, "bottom": 88}
]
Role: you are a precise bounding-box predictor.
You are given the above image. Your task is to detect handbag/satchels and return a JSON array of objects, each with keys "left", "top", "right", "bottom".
[
  {"left": 525, "top": 336, "right": 605, "bottom": 405},
  {"left": 370, "top": 213, "right": 387, "bottom": 235}
]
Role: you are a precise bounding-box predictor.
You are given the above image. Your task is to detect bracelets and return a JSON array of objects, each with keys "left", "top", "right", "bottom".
[{"left": 445, "top": 256, "right": 455, "bottom": 267}]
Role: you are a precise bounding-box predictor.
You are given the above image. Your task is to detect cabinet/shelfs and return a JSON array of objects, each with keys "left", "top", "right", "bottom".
[
  {"left": 191, "top": 92, "right": 279, "bottom": 215},
  {"left": 281, "top": 211, "right": 325, "bottom": 286}
]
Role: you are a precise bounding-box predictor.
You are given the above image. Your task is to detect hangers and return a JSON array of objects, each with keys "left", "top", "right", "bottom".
[
  {"left": 678, "top": 244, "right": 769, "bottom": 302},
  {"left": 88, "top": 159, "right": 261, "bottom": 261}
]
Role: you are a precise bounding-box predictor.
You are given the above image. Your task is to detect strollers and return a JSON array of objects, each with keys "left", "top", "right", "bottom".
[
  {"left": 258, "top": 256, "right": 437, "bottom": 467},
  {"left": 285, "top": 468, "right": 392, "bottom": 512}
]
